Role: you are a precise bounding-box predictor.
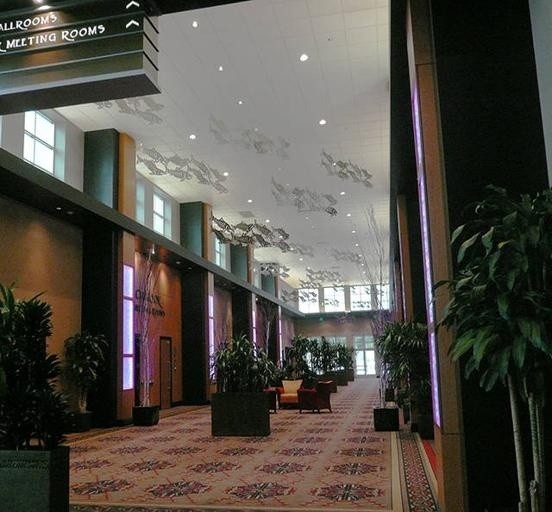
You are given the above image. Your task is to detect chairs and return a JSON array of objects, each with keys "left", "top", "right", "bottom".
[{"left": 263, "top": 378, "right": 334, "bottom": 414}]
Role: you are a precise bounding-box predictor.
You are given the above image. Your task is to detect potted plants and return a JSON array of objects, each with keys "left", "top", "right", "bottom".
[
  {"left": 372, "top": 320, "right": 436, "bottom": 441},
  {"left": 0, "top": 278, "right": 112, "bottom": 511},
  {"left": 209, "top": 330, "right": 275, "bottom": 437},
  {"left": 286, "top": 333, "right": 356, "bottom": 392}
]
